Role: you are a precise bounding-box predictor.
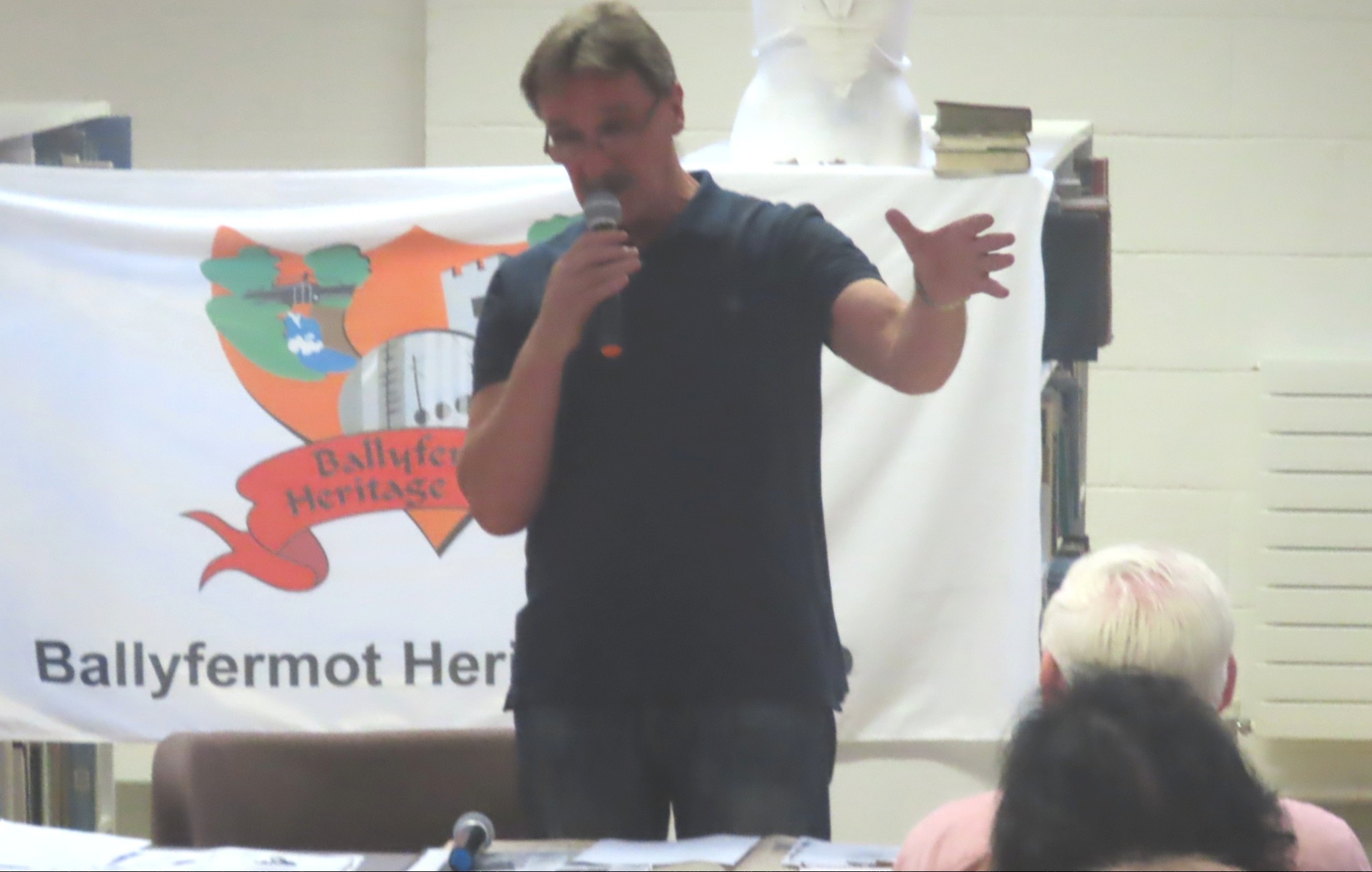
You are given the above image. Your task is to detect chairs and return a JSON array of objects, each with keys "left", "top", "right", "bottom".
[{"left": 154, "top": 728, "right": 537, "bottom": 852}]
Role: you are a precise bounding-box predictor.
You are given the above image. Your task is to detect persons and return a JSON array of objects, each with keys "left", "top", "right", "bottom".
[
  {"left": 890, "top": 541, "right": 1372, "bottom": 872},
  {"left": 456, "top": 3, "right": 1015, "bottom": 840}
]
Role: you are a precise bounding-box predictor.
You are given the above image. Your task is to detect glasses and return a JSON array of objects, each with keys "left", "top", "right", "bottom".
[{"left": 543, "top": 90, "right": 661, "bottom": 166}]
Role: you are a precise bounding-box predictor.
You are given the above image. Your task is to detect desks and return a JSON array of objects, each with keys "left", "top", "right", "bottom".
[{"left": 0, "top": 821, "right": 898, "bottom": 872}]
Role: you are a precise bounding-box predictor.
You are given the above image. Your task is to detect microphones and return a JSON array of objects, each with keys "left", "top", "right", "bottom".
[
  {"left": 583, "top": 185, "right": 625, "bottom": 356},
  {"left": 450, "top": 809, "right": 497, "bottom": 871}
]
